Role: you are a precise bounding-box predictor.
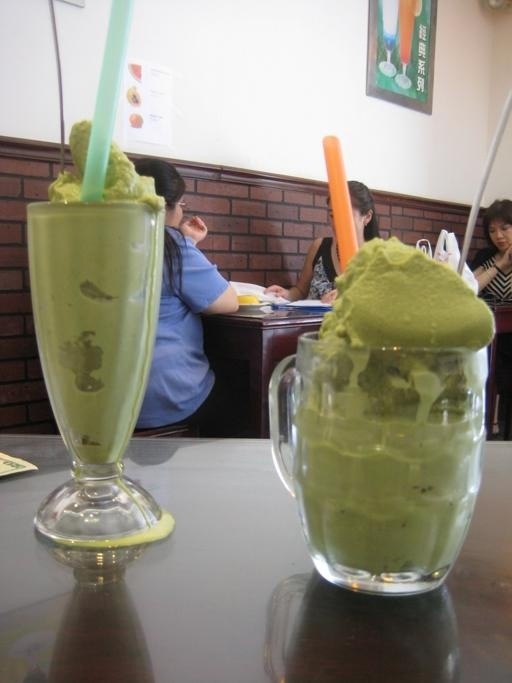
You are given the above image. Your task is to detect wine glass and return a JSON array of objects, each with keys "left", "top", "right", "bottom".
[
  {"left": 394, "top": 0, "right": 419, "bottom": 90},
  {"left": 20, "top": 194, "right": 175, "bottom": 546},
  {"left": 377, "top": 0, "right": 400, "bottom": 78}
]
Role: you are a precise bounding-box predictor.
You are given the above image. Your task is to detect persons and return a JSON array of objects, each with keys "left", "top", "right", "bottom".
[
  {"left": 126, "top": 156, "right": 253, "bottom": 439},
  {"left": 261, "top": 178, "right": 379, "bottom": 304},
  {"left": 471, "top": 194, "right": 512, "bottom": 443}
]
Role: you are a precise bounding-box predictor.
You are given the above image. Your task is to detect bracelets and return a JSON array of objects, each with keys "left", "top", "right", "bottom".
[{"left": 493, "top": 263, "right": 501, "bottom": 273}]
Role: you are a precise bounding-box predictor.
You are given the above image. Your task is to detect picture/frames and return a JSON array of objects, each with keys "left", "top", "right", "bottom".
[{"left": 364, "top": 3, "right": 439, "bottom": 118}]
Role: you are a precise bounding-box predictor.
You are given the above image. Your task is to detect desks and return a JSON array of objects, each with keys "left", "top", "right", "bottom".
[
  {"left": 198, "top": 309, "right": 324, "bottom": 442},
  {"left": 483, "top": 302, "right": 512, "bottom": 444}
]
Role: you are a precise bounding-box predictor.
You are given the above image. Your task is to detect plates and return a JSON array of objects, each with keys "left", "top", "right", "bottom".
[{"left": 238, "top": 300, "right": 270, "bottom": 310}]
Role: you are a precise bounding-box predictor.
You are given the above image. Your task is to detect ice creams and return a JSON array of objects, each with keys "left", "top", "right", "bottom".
[
  {"left": 26, "top": 121, "right": 169, "bottom": 466},
  {"left": 288, "top": 239, "right": 496, "bottom": 573}
]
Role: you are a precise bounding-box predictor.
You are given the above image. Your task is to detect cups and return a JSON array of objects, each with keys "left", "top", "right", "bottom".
[{"left": 265, "top": 328, "right": 488, "bottom": 597}]
